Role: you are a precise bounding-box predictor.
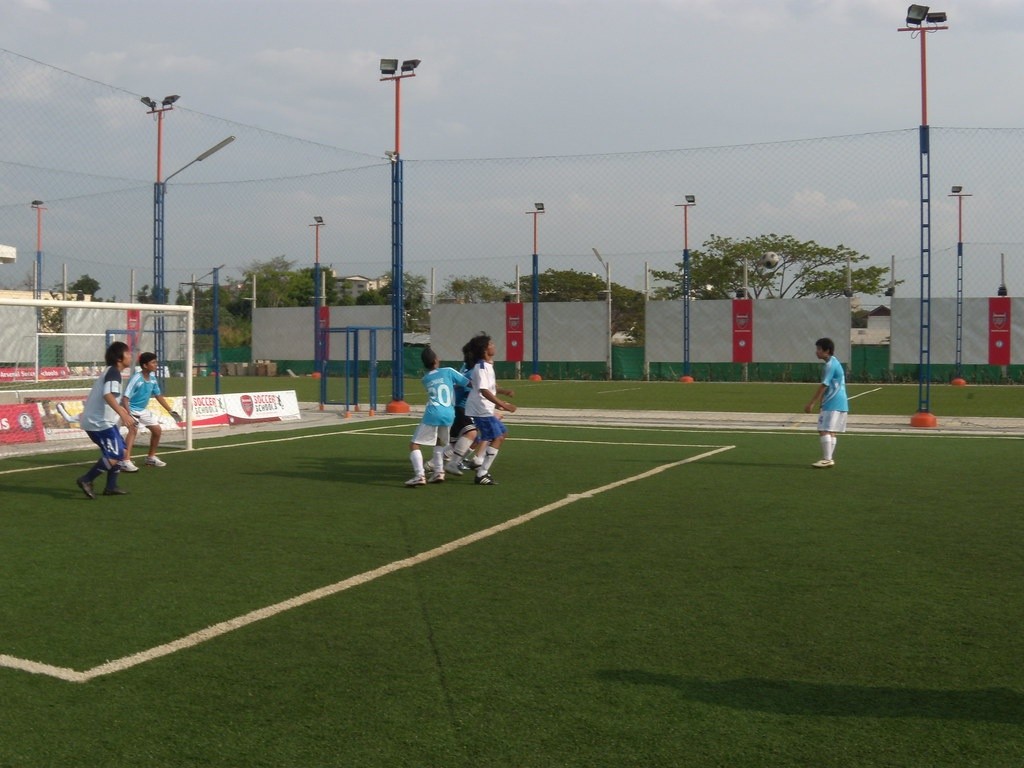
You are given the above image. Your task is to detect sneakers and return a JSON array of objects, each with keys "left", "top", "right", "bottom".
[
  {"left": 405, "top": 477, "right": 426, "bottom": 486},
  {"left": 77, "top": 476, "right": 96, "bottom": 499},
  {"left": 813, "top": 458, "right": 834, "bottom": 467},
  {"left": 428, "top": 472, "right": 446, "bottom": 482},
  {"left": 458, "top": 463, "right": 470, "bottom": 470},
  {"left": 145, "top": 457, "right": 166, "bottom": 467},
  {"left": 470, "top": 462, "right": 481, "bottom": 470},
  {"left": 475, "top": 474, "right": 497, "bottom": 485},
  {"left": 119, "top": 461, "right": 139, "bottom": 472},
  {"left": 103, "top": 487, "right": 129, "bottom": 495},
  {"left": 444, "top": 462, "right": 464, "bottom": 476},
  {"left": 424, "top": 462, "right": 435, "bottom": 471}
]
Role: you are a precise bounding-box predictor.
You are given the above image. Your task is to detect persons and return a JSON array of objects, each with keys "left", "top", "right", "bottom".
[
  {"left": 121, "top": 352, "right": 183, "bottom": 472},
  {"left": 424, "top": 363, "right": 478, "bottom": 475},
  {"left": 805, "top": 337, "right": 848, "bottom": 468},
  {"left": 405, "top": 347, "right": 472, "bottom": 487},
  {"left": 76, "top": 341, "right": 138, "bottom": 499},
  {"left": 459, "top": 328, "right": 516, "bottom": 486}
]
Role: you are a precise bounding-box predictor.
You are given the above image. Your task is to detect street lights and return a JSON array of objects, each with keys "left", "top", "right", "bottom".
[
  {"left": 896, "top": 3, "right": 950, "bottom": 425},
  {"left": 310, "top": 214, "right": 327, "bottom": 375},
  {"left": 947, "top": 185, "right": 974, "bottom": 384},
  {"left": 674, "top": 195, "right": 697, "bottom": 383},
  {"left": 139, "top": 92, "right": 239, "bottom": 391},
  {"left": 524, "top": 202, "right": 546, "bottom": 381},
  {"left": 378, "top": 58, "right": 424, "bottom": 411},
  {"left": 30, "top": 199, "right": 48, "bottom": 298}
]
